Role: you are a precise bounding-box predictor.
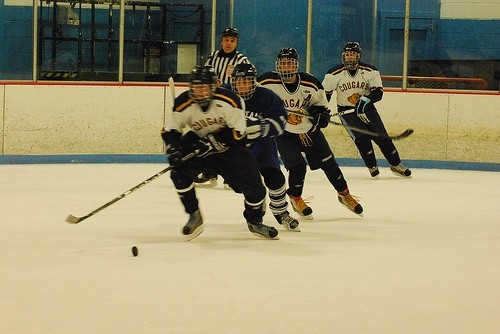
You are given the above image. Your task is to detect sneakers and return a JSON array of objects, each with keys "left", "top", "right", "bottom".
[
  {"left": 368, "top": 165, "right": 380, "bottom": 180},
  {"left": 273, "top": 210, "right": 301, "bottom": 233},
  {"left": 223, "top": 179, "right": 231, "bottom": 192},
  {"left": 338, "top": 192, "right": 364, "bottom": 217},
  {"left": 289, "top": 196, "right": 314, "bottom": 220},
  {"left": 195, "top": 175, "right": 219, "bottom": 188},
  {"left": 247, "top": 220, "right": 280, "bottom": 240},
  {"left": 182, "top": 207, "right": 205, "bottom": 242},
  {"left": 389, "top": 162, "right": 412, "bottom": 179}
]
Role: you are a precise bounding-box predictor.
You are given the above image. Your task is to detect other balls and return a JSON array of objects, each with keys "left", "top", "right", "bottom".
[{"left": 131, "top": 246, "right": 138, "bottom": 256}]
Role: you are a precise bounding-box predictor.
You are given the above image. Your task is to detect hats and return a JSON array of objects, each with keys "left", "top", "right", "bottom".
[{"left": 222, "top": 27, "right": 239, "bottom": 39}]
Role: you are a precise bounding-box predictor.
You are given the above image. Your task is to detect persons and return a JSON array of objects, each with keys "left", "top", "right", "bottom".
[
  {"left": 321, "top": 42, "right": 411, "bottom": 178},
  {"left": 161, "top": 65, "right": 280, "bottom": 242},
  {"left": 257, "top": 47, "right": 364, "bottom": 219},
  {"left": 194, "top": 28, "right": 256, "bottom": 188},
  {"left": 220, "top": 63, "right": 300, "bottom": 233}
]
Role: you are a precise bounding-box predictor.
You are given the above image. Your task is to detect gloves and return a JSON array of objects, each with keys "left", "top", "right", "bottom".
[
  {"left": 354, "top": 94, "right": 373, "bottom": 115},
  {"left": 245, "top": 115, "right": 276, "bottom": 142},
  {"left": 191, "top": 132, "right": 229, "bottom": 160},
  {"left": 166, "top": 147, "right": 185, "bottom": 172},
  {"left": 308, "top": 109, "right": 330, "bottom": 128}
]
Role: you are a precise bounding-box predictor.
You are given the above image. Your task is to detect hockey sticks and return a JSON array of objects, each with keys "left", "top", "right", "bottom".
[
  {"left": 285, "top": 107, "right": 414, "bottom": 141},
  {"left": 168, "top": 76, "right": 176, "bottom": 100},
  {"left": 64, "top": 144, "right": 209, "bottom": 225},
  {"left": 329, "top": 109, "right": 355, "bottom": 117}
]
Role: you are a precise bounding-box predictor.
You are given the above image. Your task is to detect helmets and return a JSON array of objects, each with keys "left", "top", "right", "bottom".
[
  {"left": 230, "top": 63, "right": 257, "bottom": 101},
  {"left": 341, "top": 41, "right": 362, "bottom": 70},
  {"left": 275, "top": 47, "right": 300, "bottom": 80},
  {"left": 188, "top": 64, "right": 217, "bottom": 103}
]
